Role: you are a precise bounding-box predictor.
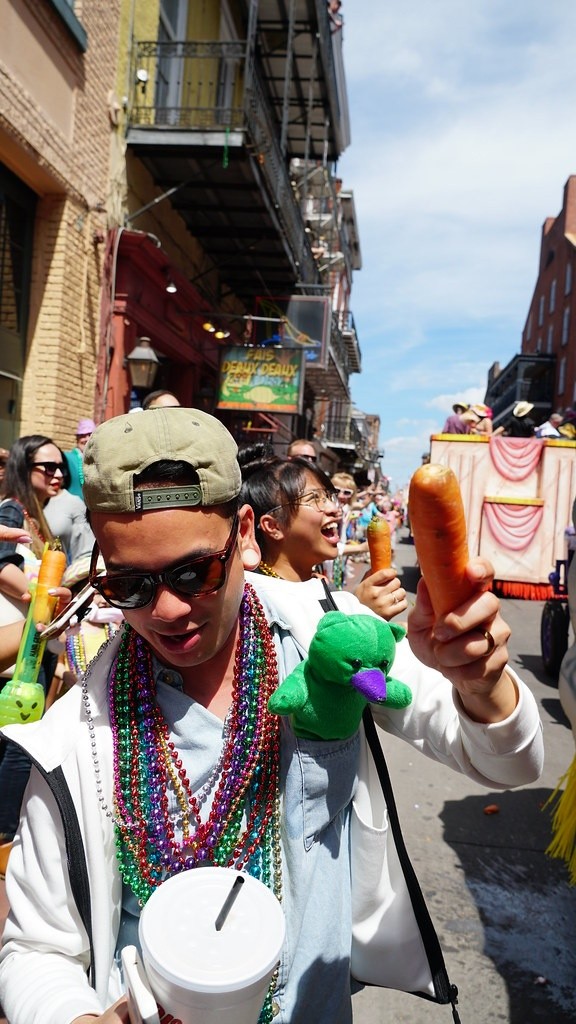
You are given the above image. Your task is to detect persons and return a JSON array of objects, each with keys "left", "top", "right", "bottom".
[
  {"left": 0, "top": 407, "right": 544, "bottom": 1024},
  {"left": 0, "top": 399, "right": 575, "bottom": 889}
]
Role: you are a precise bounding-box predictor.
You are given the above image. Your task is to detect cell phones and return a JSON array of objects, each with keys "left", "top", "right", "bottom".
[
  {"left": 40, "top": 582, "right": 97, "bottom": 640},
  {"left": 120, "top": 946, "right": 160, "bottom": 1024}
]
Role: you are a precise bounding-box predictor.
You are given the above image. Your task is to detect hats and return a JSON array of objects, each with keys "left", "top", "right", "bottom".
[
  {"left": 75, "top": 408, "right": 241, "bottom": 510},
  {"left": 74, "top": 419, "right": 95, "bottom": 435}
]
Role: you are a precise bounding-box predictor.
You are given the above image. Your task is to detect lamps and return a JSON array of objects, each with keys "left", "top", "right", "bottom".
[
  {"left": 201, "top": 316, "right": 230, "bottom": 339},
  {"left": 165, "top": 278, "right": 178, "bottom": 293},
  {"left": 122, "top": 337, "right": 160, "bottom": 388}
]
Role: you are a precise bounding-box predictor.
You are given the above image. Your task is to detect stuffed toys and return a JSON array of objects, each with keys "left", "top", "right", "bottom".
[{"left": 266, "top": 611, "right": 413, "bottom": 740}]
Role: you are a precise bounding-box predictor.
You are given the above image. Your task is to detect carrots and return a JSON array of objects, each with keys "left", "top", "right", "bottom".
[
  {"left": 367, "top": 516, "right": 392, "bottom": 574},
  {"left": 33, "top": 542, "right": 66, "bottom": 624},
  {"left": 408, "top": 463, "right": 473, "bottom": 623}
]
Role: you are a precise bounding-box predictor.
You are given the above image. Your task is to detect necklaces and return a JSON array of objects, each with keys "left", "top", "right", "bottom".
[
  {"left": 257, "top": 557, "right": 282, "bottom": 580},
  {"left": 12, "top": 496, "right": 48, "bottom": 543},
  {"left": 111, "top": 580, "right": 286, "bottom": 1024},
  {"left": 65, "top": 622, "right": 118, "bottom": 680}
]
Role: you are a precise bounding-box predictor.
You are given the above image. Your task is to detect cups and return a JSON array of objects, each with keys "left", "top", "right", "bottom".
[{"left": 138, "top": 867, "right": 285, "bottom": 1024}]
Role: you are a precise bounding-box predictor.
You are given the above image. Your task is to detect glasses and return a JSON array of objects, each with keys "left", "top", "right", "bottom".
[
  {"left": 257, "top": 489, "right": 334, "bottom": 529},
  {"left": 333, "top": 490, "right": 355, "bottom": 497},
  {"left": 87, "top": 510, "right": 237, "bottom": 610},
  {"left": 287, "top": 454, "right": 318, "bottom": 465},
  {"left": 33, "top": 462, "right": 63, "bottom": 477}
]
